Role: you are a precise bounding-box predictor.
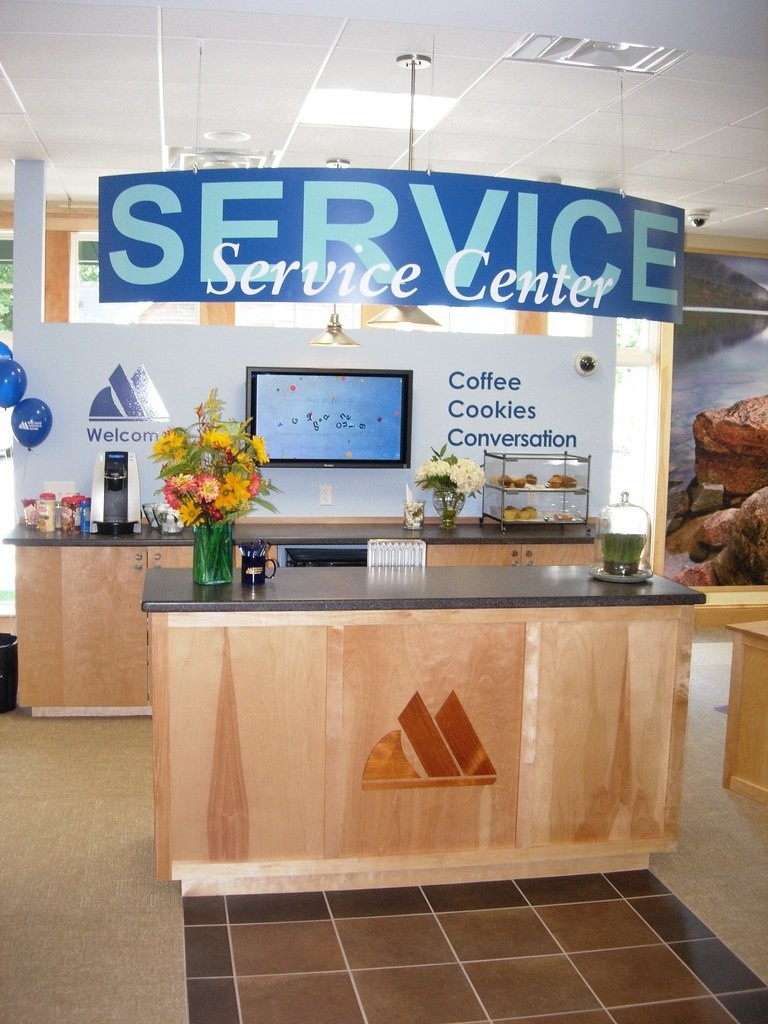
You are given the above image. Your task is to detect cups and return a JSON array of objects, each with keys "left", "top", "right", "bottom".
[
  {"left": 241, "top": 555, "right": 276, "bottom": 584},
  {"left": 21, "top": 498, "right": 37, "bottom": 525},
  {"left": 403, "top": 499, "right": 425, "bottom": 530}
]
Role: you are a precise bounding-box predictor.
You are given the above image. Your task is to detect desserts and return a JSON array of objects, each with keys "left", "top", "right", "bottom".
[
  {"left": 503, "top": 505, "right": 538, "bottom": 521},
  {"left": 496, "top": 474, "right": 578, "bottom": 490}
]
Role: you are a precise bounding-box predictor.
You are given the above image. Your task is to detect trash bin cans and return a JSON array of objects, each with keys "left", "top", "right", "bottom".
[{"left": 0, "top": 633, "right": 17, "bottom": 715}]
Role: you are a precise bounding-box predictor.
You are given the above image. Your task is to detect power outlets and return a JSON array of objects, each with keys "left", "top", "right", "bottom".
[{"left": 319, "top": 484, "right": 332, "bottom": 506}]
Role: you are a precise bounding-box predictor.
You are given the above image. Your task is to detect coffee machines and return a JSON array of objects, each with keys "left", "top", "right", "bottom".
[{"left": 93, "top": 451, "right": 139, "bottom": 534}]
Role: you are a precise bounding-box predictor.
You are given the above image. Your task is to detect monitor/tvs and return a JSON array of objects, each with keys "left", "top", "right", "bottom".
[{"left": 245, "top": 367, "right": 413, "bottom": 470}]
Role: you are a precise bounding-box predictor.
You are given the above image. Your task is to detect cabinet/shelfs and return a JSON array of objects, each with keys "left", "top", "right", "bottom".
[
  {"left": 137, "top": 555, "right": 710, "bottom": 899},
  {"left": 428, "top": 546, "right": 602, "bottom": 569},
  {"left": 721, "top": 616, "right": 768, "bottom": 809},
  {"left": 482, "top": 447, "right": 591, "bottom": 524},
  {"left": 12, "top": 547, "right": 278, "bottom": 716}
]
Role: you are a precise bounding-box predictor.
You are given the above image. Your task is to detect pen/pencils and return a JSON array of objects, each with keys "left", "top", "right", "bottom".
[{"left": 240, "top": 540, "right": 271, "bottom": 557}]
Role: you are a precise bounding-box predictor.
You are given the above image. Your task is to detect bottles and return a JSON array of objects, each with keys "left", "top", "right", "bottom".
[
  {"left": 37, "top": 492, "right": 61, "bottom": 532},
  {"left": 61, "top": 496, "right": 91, "bottom": 532}
]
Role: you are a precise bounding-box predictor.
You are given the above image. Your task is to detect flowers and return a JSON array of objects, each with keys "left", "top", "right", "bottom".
[
  {"left": 150, "top": 386, "right": 285, "bottom": 531},
  {"left": 411, "top": 447, "right": 487, "bottom": 527}
]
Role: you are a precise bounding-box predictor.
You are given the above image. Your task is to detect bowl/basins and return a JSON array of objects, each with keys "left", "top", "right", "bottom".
[{"left": 141, "top": 503, "right": 184, "bottom": 534}]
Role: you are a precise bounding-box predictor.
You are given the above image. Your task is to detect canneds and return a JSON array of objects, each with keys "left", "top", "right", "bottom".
[{"left": 38, "top": 494, "right": 92, "bottom": 534}]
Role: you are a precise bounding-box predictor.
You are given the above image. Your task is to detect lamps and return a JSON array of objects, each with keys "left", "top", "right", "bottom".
[
  {"left": 366, "top": 53, "right": 443, "bottom": 332},
  {"left": 305, "top": 155, "right": 361, "bottom": 351}
]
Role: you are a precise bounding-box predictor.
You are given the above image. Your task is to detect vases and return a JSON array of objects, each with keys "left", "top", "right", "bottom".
[
  {"left": 191, "top": 522, "right": 235, "bottom": 587},
  {"left": 432, "top": 487, "right": 465, "bottom": 530}
]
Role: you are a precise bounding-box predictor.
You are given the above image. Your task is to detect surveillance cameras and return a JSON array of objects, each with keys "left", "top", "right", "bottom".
[
  {"left": 575, "top": 352, "right": 599, "bottom": 377},
  {"left": 687, "top": 214, "right": 710, "bottom": 227}
]
